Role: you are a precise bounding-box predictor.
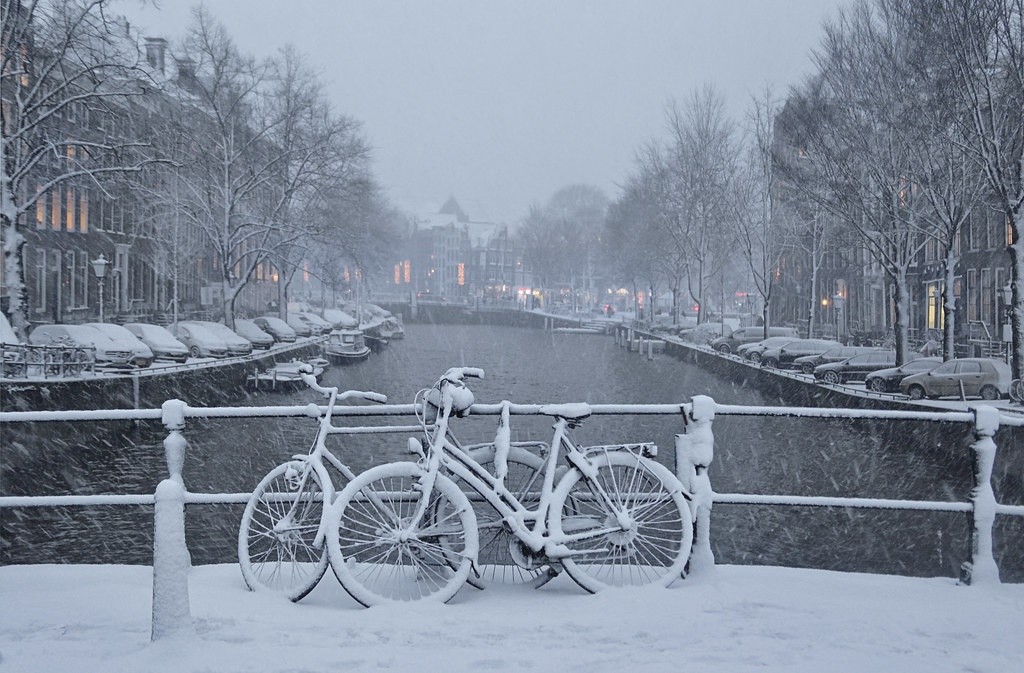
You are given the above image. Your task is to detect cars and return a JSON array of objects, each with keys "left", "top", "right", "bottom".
[
  {"left": 899, "top": 357, "right": 1011, "bottom": 402},
  {"left": 167, "top": 323, "right": 229, "bottom": 361},
  {"left": 414, "top": 290, "right": 446, "bottom": 303},
  {"left": 219, "top": 318, "right": 275, "bottom": 351},
  {"left": 263, "top": 301, "right": 392, "bottom": 338},
  {"left": 123, "top": 323, "right": 190, "bottom": 364},
  {"left": 865, "top": 359, "right": 943, "bottom": 393}
]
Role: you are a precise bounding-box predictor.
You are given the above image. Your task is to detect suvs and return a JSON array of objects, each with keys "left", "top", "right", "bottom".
[{"left": 83, "top": 322, "right": 154, "bottom": 369}]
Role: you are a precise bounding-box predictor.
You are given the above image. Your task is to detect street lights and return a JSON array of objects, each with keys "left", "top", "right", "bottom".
[
  {"left": 90, "top": 253, "right": 112, "bottom": 322},
  {"left": 832, "top": 290, "right": 843, "bottom": 343},
  {"left": 747, "top": 291, "right": 755, "bottom": 326},
  {"left": 996, "top": 279, "right": 1014, "bottom": 366}
]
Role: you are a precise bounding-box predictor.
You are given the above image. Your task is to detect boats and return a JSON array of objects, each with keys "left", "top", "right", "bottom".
[
  {"left": 320, "top": 328, "right": 373, "bottom": 366},
  {"left": 385, "top": 319, "right": 404, "bottom": 339},
  {"left": 243, "top": 357, "right": 325, "bottom": 394}
]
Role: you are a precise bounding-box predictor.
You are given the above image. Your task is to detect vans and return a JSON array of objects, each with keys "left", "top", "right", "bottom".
[
  {"left": 641, "top": 313, "right": 915, "bottom": 386},
  {"left": 247, "top": 317, "right": 297, "bottom": 345},
  {"left": 30, "top": 324, "right": 136, "bottom": 375},
  {"left": 0, "top": 310, "right": 24, "bottom": 373},
  {"left": 181, "top": 320, "right": 253, "bottom": 358}
]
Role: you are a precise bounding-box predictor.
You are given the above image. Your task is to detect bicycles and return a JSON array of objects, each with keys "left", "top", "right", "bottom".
[
  {"left": 1008, "top": 376, "right": 1024, "bottom": 403},
  {"left": 239, "top": 363, "right": 694, "bottom": 608},
  {"left": 5, "top": 332, "right": 84, "bottom": 378}
]
